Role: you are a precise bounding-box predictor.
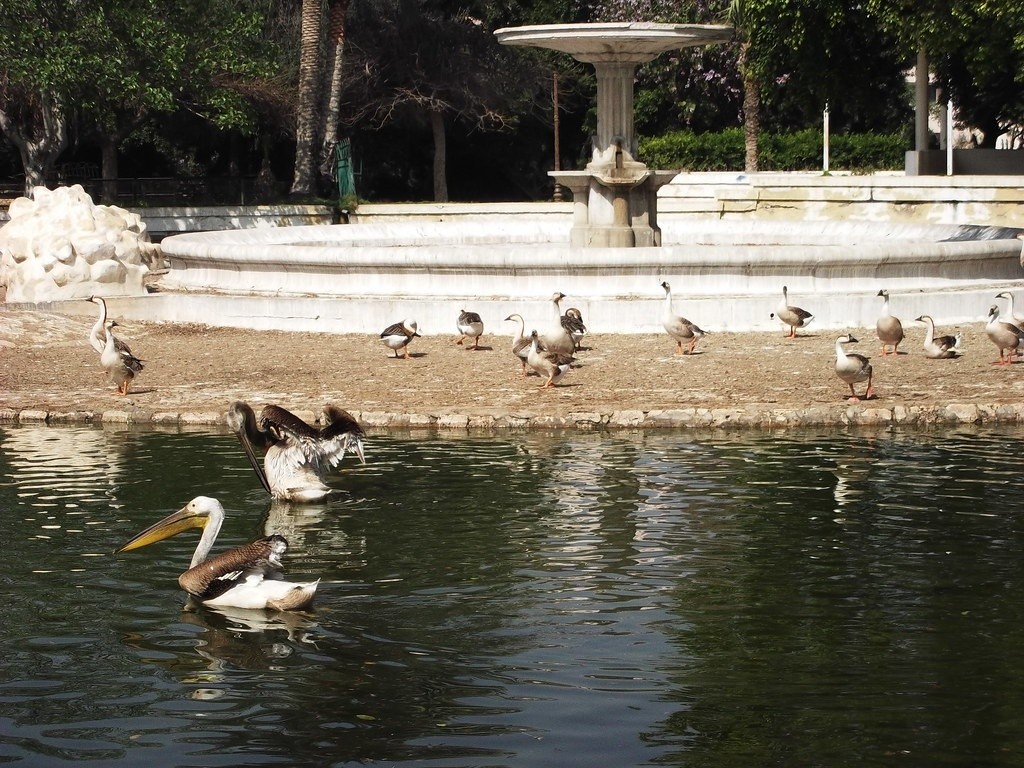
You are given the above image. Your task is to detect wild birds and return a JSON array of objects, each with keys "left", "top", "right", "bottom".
[
  {"left": 87, "top": 295, "right": 147, "bottom": 395},
  {"left": 985, "top": 234, "right": 1024, "bottom": 365},
  {"left": 377, "top": 318, "right": 421, "bottom": 358},
  {"left": 876, "top": 290, "right": 904, "bottom": 357},
  {"left": 835, "top": 333, "right": 874, "bottom": 403},
  {"left": 503, "top": 291, "right": 586, "bottom": 389},
  {"left": 916, "top": 315, "right": 967, "bottom": 359},
  {"left": 777, "top": 287, "right": 815, "bottom": 339},
  {"left": 226, "top": 399, "right": 366, "bottom": 502},
  {"left": 456, "top": 309, "right": 483, "bottom": 350},
  {"left": 113, "top": 495, "right": 321, "bottom": 612},
  {"left": 661, "top": 283, "right": 709, "bottom": 355}
]
